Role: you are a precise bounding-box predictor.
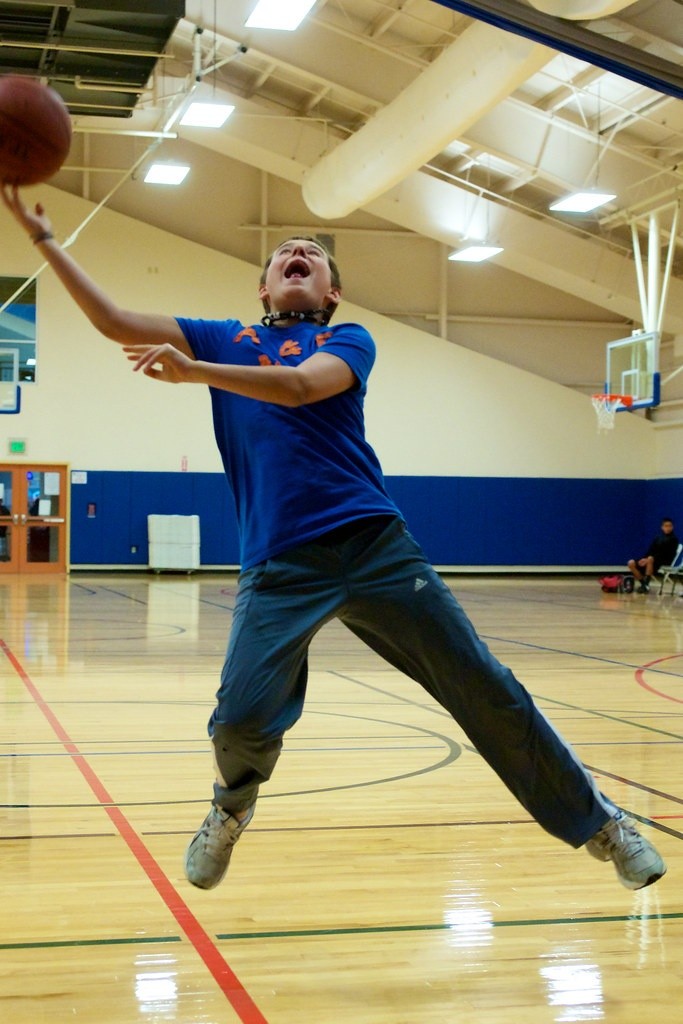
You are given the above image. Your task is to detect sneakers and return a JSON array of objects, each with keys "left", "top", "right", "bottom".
[
  {"left": 185, "top": 795, "right": 256, "bottom": 890},
  {"left": 584, "top": 810, "right": 667, "bottom": 889}
]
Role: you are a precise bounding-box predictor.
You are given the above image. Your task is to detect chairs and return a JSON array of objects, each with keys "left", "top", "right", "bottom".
[{"left": 656, "top": 544, "right": 683, "bottom": 601}]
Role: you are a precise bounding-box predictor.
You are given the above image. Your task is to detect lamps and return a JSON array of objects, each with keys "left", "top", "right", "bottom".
[
  {"left": 180, "top": 0, "right": 236, "bottom": 128},
  {"left": 244, "top": 0, "right": 317, "bottom": 31},
  {"left": 548, "top": 79, "right": 620, "bottom": 213},
  {"left": 448, "top": 157, "right": 503, "bottom": 264},
  {"left": 144, "top": 158, "right": 192, "bottom": 185}
]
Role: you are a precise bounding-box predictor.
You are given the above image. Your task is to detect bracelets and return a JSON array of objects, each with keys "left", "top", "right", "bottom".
[{"left": 30, "top": 231, "right": 53, "bottom": 244}]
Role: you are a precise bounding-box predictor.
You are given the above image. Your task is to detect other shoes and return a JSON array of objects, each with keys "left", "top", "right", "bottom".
[{"left": 637, "top": 586, "right": 649, "bottom": 593}]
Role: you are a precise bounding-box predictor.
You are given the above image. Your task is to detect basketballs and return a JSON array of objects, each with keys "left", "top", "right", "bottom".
[{"left": 1, "top": 70, "right": 75, "bottom": 189}]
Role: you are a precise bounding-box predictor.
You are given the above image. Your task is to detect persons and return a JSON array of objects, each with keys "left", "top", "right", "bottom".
[
  {"left": 628, "top": 520, "right": 678, "bottom": 593},
  {"left": 0, "top": 183, "right": 666, "bottom": 891},
  {"left": 0, "top": 498, "right": 10, "bottom": 557}
]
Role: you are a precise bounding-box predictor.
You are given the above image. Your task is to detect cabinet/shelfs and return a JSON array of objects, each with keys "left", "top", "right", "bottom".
[{"left": 146, "top": 514, "right": 201, "bottom": 573}]
